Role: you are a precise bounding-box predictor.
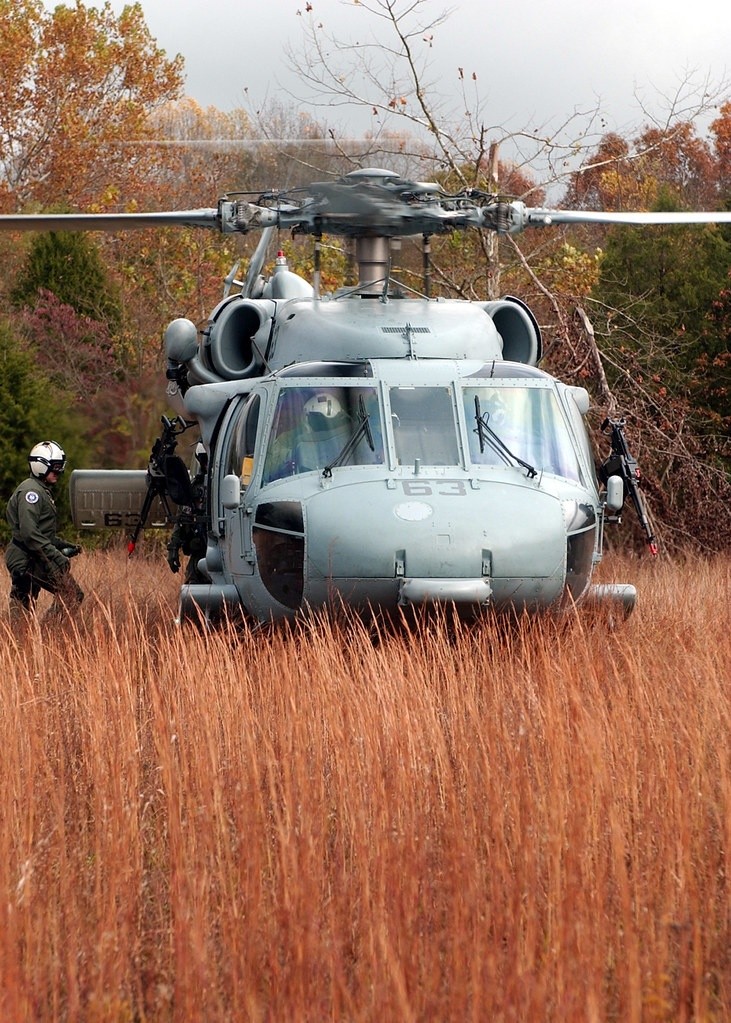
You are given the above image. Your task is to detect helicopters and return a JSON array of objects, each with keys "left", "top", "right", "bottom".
[{"left": 0, "top": 169, "right": 731, "bottom": 650}]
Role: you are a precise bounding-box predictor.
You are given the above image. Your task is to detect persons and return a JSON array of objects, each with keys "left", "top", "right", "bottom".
[
  {"left": 5, "top": 440, "right": 84, "bottom": 624},
  {"left": 151, "top": 442, "right": 210, "bottom": 584},
  {"left": 470, "top": 387, "right": 641, "bottom": 505},
  {"left": 263, "top": 393, "right": 381, "bottom": 485}
]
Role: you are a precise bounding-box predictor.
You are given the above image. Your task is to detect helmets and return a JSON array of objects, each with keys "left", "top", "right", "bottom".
[
  {"left": 29, "top": 440, "right": 66, "bottom": 478},
  {"left": 301, "top": 393, "right": 343, "bottom": 436}
]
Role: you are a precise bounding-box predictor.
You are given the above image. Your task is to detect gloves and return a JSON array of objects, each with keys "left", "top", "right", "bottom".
[
  {"left": 42, "top": 544, "right": 71, "bottom": 575},
  {"left": 58, "top": 542, "right": 78, "bottom": 558},
  {"left": 338, "top": 408, "right": 354, "bottom": 423}
]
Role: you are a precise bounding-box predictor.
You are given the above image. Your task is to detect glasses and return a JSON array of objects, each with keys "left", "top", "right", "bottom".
[{"left": 51, "top": 462, "right": 65, "bottom": 473}]
